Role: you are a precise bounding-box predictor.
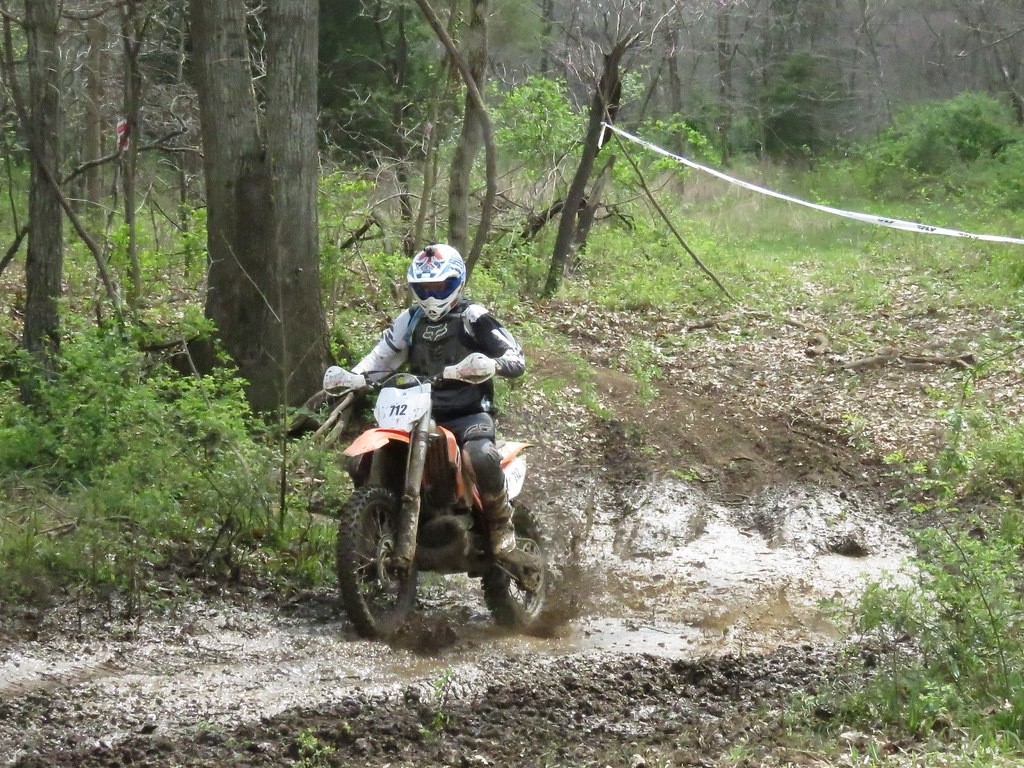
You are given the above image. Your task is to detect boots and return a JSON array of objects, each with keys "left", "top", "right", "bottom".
[{"left": 476, "top": 475, "right": 516, "bottom": 557}]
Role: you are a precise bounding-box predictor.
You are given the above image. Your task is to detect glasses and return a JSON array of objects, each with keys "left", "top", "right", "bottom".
[{"left": 411, "top": 278, "right": 461, "bottom": 300}]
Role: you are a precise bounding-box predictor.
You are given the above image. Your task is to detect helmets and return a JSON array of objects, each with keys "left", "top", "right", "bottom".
[{"left": 407, "top": 243, "right": 466, "bottom": 321}]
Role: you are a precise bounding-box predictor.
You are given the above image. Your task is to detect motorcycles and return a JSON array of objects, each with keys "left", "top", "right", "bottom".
[{"left": 325, "top": 353, "right": 551, "bottom": 644}]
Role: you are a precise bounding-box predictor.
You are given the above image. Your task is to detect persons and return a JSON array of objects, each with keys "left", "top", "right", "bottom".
[{"left": 322, "top": 243, "right": 526, "bottom": 560}]
